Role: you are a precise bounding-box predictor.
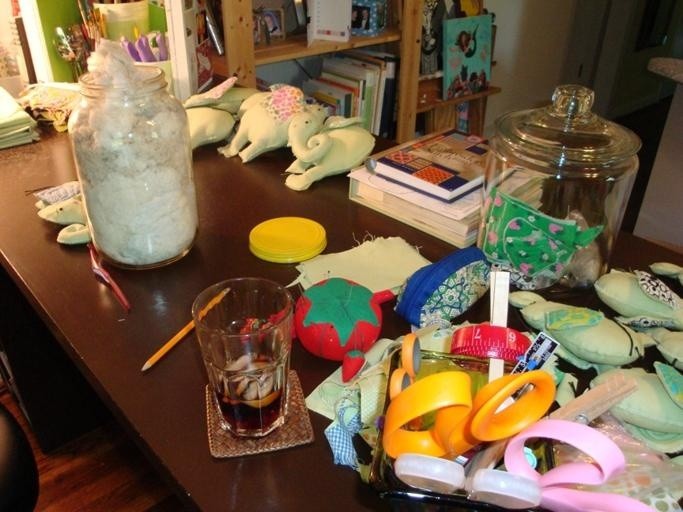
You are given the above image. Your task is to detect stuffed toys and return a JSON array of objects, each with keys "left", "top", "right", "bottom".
[
  {"left": 35, "top": 174, "right": 92, "bottom": 255},
  {"left": 518, "top": 262, "right": 683, "bottom": 447},
  {"left": 289, "top": 266, "right": 382, "bottom": 381},
  {"left": 169, "top": 83, "right": 369, "bottom": 187}
]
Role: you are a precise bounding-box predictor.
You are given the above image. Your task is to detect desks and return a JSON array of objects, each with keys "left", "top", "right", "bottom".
[{"left": 1, "top": 86, "right": 682, "bottom": 512}]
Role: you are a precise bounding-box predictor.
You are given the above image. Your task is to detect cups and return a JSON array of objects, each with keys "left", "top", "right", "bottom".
[{"left": 191, "top": 276, "right": 291, "bottom": 441}]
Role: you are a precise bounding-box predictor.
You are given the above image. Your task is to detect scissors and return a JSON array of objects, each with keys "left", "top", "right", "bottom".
[
  {"left": 380, "top": 371, "right": 658, "bottom": 512},
  {"left": 389, "top": 334, "right": 422, "bottom": 403}
]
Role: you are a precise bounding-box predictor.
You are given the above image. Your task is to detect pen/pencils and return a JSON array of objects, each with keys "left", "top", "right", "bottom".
[
  {"left": 141, "top": 287, "right": 231, "bottom": 372},
  {"left": 78, "top": 0, "right": 107, "bottom": 44}
]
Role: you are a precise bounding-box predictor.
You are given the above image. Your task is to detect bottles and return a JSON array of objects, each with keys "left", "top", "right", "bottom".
[
  {"left": 63, "top": 68, "right": 204, "bottom": 275},
  {"left": 476, "top": 83, "right": 644, "bottom": 304}
]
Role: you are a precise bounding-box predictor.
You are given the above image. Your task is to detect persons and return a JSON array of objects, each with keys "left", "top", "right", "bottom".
[
  {"left": 360, "top": 9, "right": 369, "bottom": 30},
  {"left": 351, "top": 10, "right": 358, "bottom": 27}
]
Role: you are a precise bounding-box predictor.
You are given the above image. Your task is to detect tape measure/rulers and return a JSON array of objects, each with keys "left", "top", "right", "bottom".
[{"left": 451, "top": 325, "right": 531, "bottom": 362}]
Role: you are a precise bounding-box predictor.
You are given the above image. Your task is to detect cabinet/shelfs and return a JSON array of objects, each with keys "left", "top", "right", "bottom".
[
  {"left": 413, "top": 0, "right": 504, "bottom": 144},
  {"left": 195, "top": 1, "right": 429, "bottom": 152}
]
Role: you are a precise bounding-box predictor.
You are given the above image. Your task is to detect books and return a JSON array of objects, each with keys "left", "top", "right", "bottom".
[
  {"left": 0, "top": 86, "right": 36, "bottom": 148},
  {"left": 352, "top": 127, "right": 532, "bottom": 246},
  {"left": 299, "top": 47, "right": 400, "bottom": 140},
  {"left": 444, "top": 17, "right": 490, "bottom": 97}
]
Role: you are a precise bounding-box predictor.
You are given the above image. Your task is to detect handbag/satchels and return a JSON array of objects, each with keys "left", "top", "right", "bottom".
[{"left": 393, "top": 246, "right": 491, "bottom": 329}]
